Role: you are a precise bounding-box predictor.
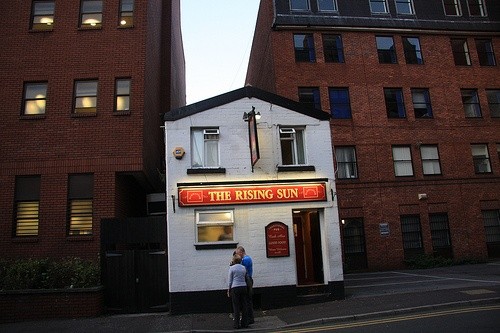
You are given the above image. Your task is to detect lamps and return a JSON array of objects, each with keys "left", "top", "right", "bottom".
[{"left": 254, "top": 112, "right": 261, "bottom": 119}]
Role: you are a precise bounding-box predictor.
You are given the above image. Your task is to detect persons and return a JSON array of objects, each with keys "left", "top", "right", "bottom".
[
  {"left": 229, "top": 250, "right": 243, "bottom": 321},
  {"left": 236, "top": 246, "right": 254, "bottom": 324},
  {"left": 217, "top": 225, "right": 232, "bottom": 241},
  {"left": 227, "top": 254, "right": 249, "bottom": 330}
]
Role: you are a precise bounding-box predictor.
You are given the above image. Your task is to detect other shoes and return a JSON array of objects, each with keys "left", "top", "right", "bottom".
[{"left": 228, "top": 313, "right": 256, "bottom": 329}]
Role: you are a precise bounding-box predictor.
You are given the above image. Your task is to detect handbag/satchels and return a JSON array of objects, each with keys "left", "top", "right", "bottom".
[{"left": 245, "top": 273, "right": 253, "bottom": 288}]
